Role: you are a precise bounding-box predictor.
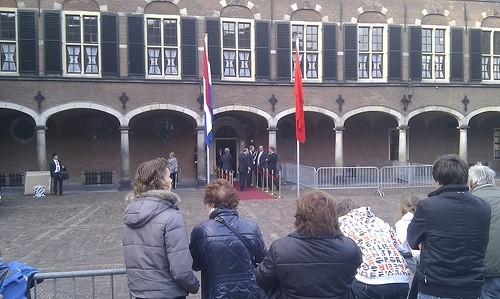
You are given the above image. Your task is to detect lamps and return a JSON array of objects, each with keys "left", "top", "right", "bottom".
[{"left": 406, "top": 78, "right": 415, "bottom": 103}]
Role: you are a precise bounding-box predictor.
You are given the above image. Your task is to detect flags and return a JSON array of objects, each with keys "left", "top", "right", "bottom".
[
  {"left": 294, "top": 48, "right": 306, "bottom": 143},
  {"left": 203, "top": 36, "right": 214, "bottom": 147}
]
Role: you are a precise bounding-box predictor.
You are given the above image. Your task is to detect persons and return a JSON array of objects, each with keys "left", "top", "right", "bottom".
[
  {"left": 254, "top": 146, "right": 269, "bottom": 187},
  {"left": 265, "top": 147, "right": 279, "bottom": 191},
  {"left": 395, "top": 193, "right": 422, "bottom": 299},
  {"left": 50, "top": 153, "right": 64, "bottom": 196},
  {"left": 122, "top": 158, "right": 201, "bottom": 299},
  {"left": 218, "top": 148, "right": 233, "bottom": 173},
  {"left": 249, "top": 145, "right": 258, "bottom": 187},
  {"left": 467, "top": 162, "right": 500, "bottom": 299},
  {"left": 336, "top": 198, "right": 413, "bottom": 299},
  {"left": 166, "top": 152, "right": 178, "bottom": 189},
  {"left": 293, "top": 32, "right": 300, "bottom": 49},
  {"left": 407, "top": 154, "right": 491, "bottom": 299},
  {"left": 256, "top": 189, "right": 363, "bottom": 299},
  {"left": 238, "top": 148, "right": 251, "bottom": 191},
  {"left": 189, "top": 179, "right": 269, "bottom": 299}
]
